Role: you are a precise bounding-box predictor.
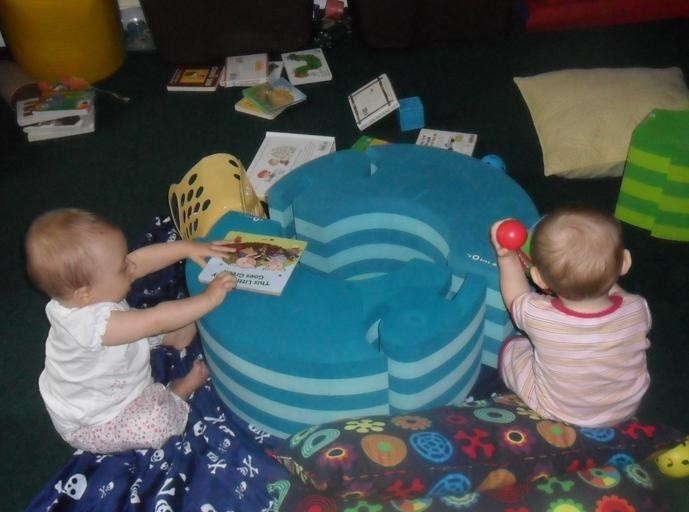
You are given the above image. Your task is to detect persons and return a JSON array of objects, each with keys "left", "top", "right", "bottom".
[
  {"left": 24, "top": 207, "right": 237, "bottom": 455},
  {"left": 490, "top": 207, "right": 653, "bottom": 428}
]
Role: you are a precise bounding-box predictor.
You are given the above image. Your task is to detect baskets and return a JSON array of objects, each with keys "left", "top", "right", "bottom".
[{"left": 168, "top": 153, "right": 266, "bottom": 244}]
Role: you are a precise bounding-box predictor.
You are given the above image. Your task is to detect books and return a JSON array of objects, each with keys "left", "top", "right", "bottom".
[
  {"left": 352, "top": 136, "right": 392, "bottom": 151},
  {"left": 247, "top": 131, "right": 336, "bottom": 201},
  {"left": 414, "top": 129, "right": 477, "bottom": 156},
  {"left": 165, "top": 47, "right": 332, "bottom": 120},
  {"left": 196, "top": 229, "right": 308, "bottom": 298},
  {"left": 16, "top": 89, "right": 99, "bottom": 143},
  {"left": 348, "top": 72, "right": 399, "bottom": 131}
]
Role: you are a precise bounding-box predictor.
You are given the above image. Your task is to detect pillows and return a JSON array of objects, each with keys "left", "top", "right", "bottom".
[
  {"left": 260, "top": 389, "right": 688, "bottom": 512},
  {"left": 514, "top": 62, "right": 687, "bottom": 180}
]
